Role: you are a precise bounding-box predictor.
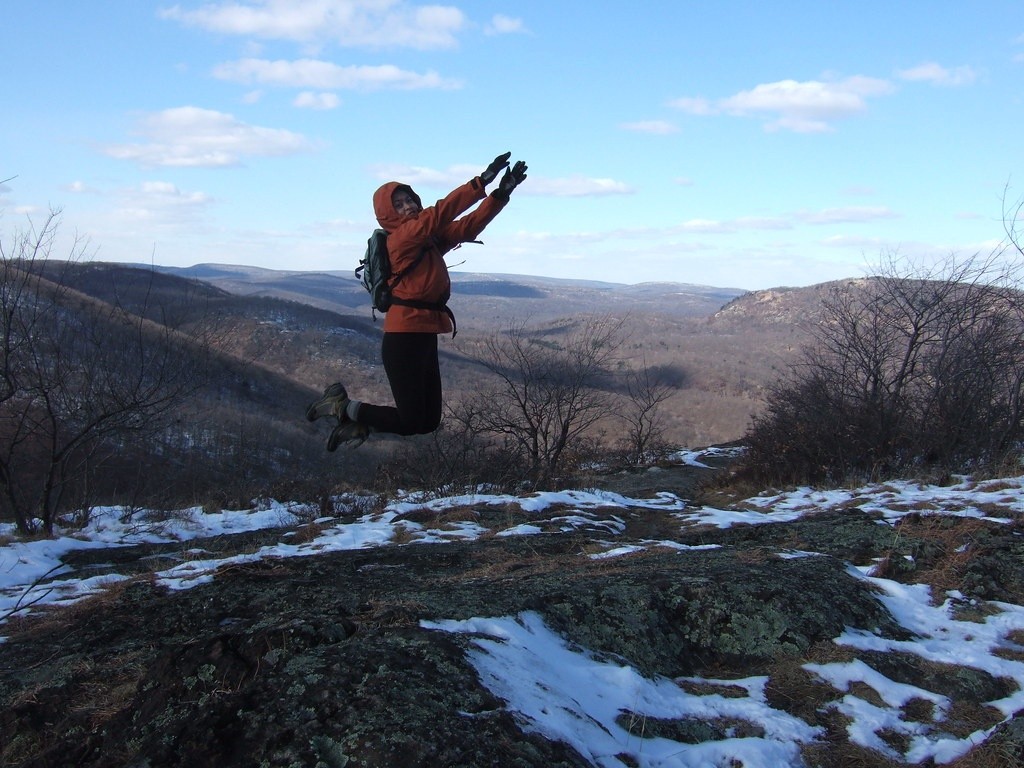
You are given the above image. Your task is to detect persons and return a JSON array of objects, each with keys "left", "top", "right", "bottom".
[{"left": 306, "top": 153, "right": 528, "bottom": 451}]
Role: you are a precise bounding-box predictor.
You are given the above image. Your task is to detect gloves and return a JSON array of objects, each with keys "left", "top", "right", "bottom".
[
  {"left": 500, "top": 161, "right": 528, "bottom": 195},
  {"left": 481, "top": 152, "right": 511, "bottom": 186}
]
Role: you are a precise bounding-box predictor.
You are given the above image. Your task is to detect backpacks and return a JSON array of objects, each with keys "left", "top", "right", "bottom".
[{"left": 356, "top": 229, "right": 450, "bottom": 312}]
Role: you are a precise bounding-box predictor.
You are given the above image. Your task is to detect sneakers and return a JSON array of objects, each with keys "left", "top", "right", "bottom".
[
  {"left": 327, "top": 421, "right": 370, "bottom": 453},
  {"left": 305, "top": 382, "right": 351, "bottom": 423}
]
